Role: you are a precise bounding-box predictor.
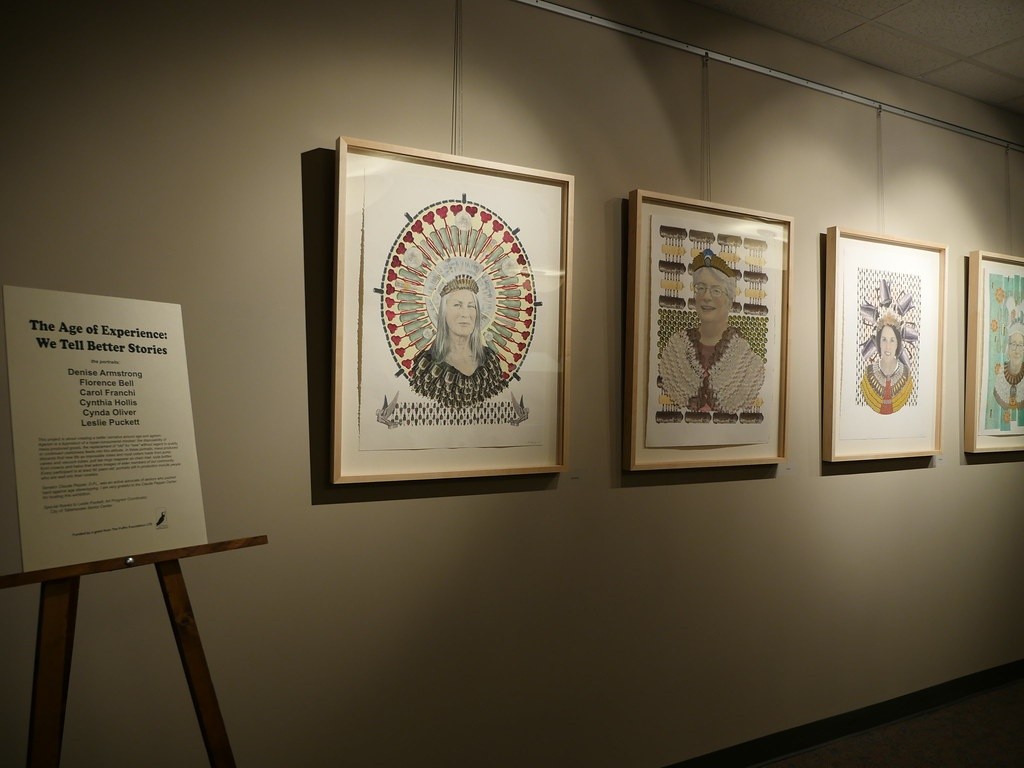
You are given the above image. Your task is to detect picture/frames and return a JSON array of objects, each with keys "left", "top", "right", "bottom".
[
  {"left": 823, "top": 226, "right": 948, "bottom": 464},
  {"left": 965, "top": 251, "right": 1024, "bottom": 456},
  {"left": 317, "top": 135, "right": 577, "bottom": 485},
  {"left": 624, "top": 188, "right": 790, "bottom": 475}
]
